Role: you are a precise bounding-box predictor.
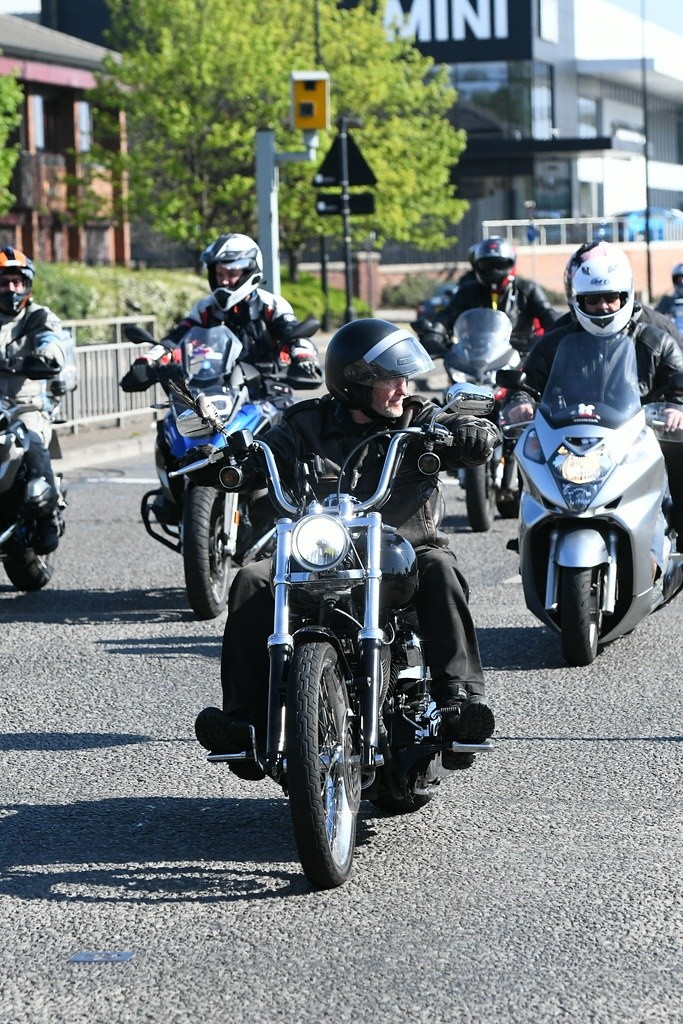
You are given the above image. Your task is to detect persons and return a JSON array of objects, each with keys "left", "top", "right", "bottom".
[
  {"left": 0, "top": 246, "right": 65, "bottom": 555},
  {"left": 177, "top": 317, "right": 498, "bottom": 780},
  {"left": 405, "top": 237, "right": 683, "bottom": 556},
  {"left": 119, "top": 231, "right": 325, "bottom": 528}
]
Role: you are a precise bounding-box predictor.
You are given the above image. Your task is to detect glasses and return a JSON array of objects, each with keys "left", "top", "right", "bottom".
[{"left": 586, "top": 292, "right": 620, "bottom": 305}]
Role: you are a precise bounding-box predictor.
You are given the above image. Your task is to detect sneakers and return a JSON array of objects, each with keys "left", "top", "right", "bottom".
[
  {"left": 196, "top": 707, "right": 266, "bottom": 781},
  {"left": 442, "top": 694, "right": 494, "bottom": 770}
]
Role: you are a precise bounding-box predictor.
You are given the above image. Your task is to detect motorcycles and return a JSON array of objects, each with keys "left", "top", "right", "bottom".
[
  {"left": 0, "top": 357, "right": 67, "bottom": 592},
  {"left": 123, "top": 319, "right": 321, "bottom": 620},
  {"left": 152, "top": 367, "right": 505, "bottom": 890},
  {"left": 499, "top": 332, "right": 683, "bottom": 666},
  {"left": 409, "top": 307, "right": 543, "bottom": 533},
  {"left": 666, "top": 298, "right": 683, "bottom": 332}
]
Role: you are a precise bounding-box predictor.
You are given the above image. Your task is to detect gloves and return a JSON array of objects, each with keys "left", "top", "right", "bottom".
[
  {"left": 23, "top": 350, "right": 58, "bottom": 380},
  {"left": 122, "top": 363, "right": 154, "bottom": 393},
  {"left": 288, "top": 360, "right": 322, "bottom": 379},
  {"left": 419, "top": 323, "right": 446, "bottom": 356},
  {"left": 182, "top": 443, "right": 224, "bottom": 487},
  {"left": 453, "top": 421, "right": 497, "bottom": 462}
]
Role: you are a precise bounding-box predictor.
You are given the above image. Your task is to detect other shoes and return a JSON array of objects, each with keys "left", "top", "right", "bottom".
[{"left": 32, "top": 515, "right": 60, "bottom": 555}]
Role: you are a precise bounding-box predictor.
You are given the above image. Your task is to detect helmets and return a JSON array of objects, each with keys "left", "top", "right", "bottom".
[
  {"left": 0, "top": 245, "right": 36, "bottom": 314},
  {"left": 564, "top": 240, "right": 634, "bottom": 337},
  {"left": 200, "top": 233, "right": 263, "bottom": 311},
  {"left": 672, "top": 264, "right": 683, "bottom": 298},
  {"left": 324, "top": 317, "right": 435, "bottom": 408},
  {"left": 467, "top": 235, "right": 516, "bottom": 293}
]
